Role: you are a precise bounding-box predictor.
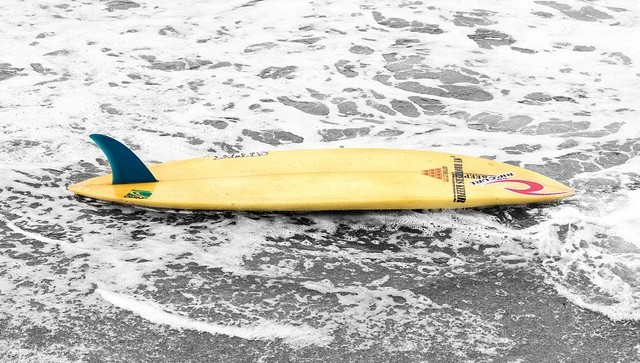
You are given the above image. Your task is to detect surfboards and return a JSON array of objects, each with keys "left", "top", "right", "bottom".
[{"left": 68, "top": 134, "right": 575, "bottom": 212}]
